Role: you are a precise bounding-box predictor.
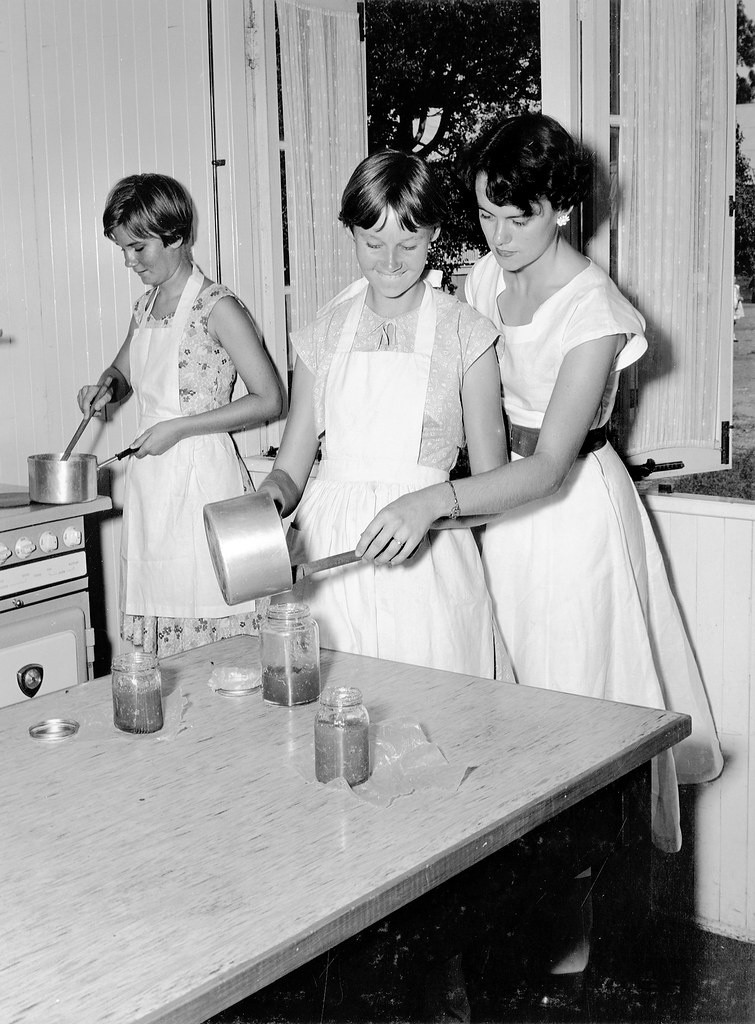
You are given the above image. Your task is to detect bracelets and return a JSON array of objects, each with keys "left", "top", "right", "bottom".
[{"left": 445, "top": 481, "right": 460, "bottom": 520}]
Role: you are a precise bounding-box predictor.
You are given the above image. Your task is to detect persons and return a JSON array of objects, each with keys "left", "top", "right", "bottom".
[
  {"left": 749, "top": 275, "right": 755, "bottom": 303},
  {"left": 733, "top": 276, "right": 745, "bottom": 342},
  {"left": 256, "top": 149, "right": 519, "bottom": 1024},
  {"left": 77, "top": 172, "right": 282, "bottom": 659},
  {"left": 354, "top": 114, "right": 725, "bottom": 974}
]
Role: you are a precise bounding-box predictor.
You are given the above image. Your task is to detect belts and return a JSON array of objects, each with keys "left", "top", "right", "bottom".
[{"left": 505, "top": 416, "right": 606, "bottom": 463}]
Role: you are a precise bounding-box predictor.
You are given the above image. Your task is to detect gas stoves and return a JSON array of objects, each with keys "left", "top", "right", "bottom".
[{"left": 0, "top": 483, "right": 120, "bottom": 570}]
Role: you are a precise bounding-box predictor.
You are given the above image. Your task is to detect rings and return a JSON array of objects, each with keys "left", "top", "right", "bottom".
[{"left": 394, "top": 539, "right": 403, "bottom": 546}]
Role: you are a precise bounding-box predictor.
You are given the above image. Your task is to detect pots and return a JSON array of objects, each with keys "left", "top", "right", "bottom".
[
  {"left": 27, "top": 447, "right": 140, "bottom": 505},
  {"left": 200, "top": 491, "right": 297, "bottom": 606}
]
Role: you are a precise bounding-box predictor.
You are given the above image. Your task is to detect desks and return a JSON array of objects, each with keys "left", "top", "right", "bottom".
[{"left": 0, "top": 635, "right": 691, "bottom": 1024}]
